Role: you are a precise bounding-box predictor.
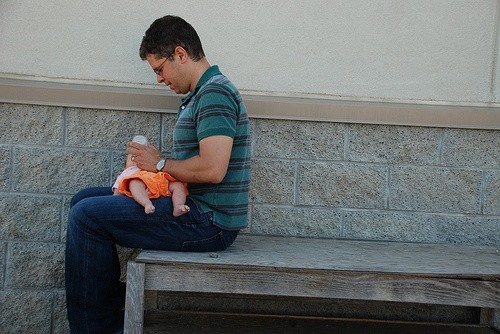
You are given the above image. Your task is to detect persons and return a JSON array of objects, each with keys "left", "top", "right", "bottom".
[
  {"left": 64, "top": 14, "right": 252, "bottom": 334},
  {"left": 112, "top": 152, "right": 191, "bottom": 217}
]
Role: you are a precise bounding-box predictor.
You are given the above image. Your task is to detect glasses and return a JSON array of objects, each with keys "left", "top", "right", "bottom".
[{"left": 153, "top": 49, "right": 175, "bottom": 75}]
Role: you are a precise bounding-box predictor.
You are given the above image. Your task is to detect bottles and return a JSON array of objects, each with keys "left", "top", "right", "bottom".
[{"left": 133, "top": 135, "right": 149, "bottom": 147}]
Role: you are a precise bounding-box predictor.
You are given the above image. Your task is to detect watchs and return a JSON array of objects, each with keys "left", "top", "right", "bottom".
[{"left": 156, "top": 157, "right": 170, "bottom": 172}]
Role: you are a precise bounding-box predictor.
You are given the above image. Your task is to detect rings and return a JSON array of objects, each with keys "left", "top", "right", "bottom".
[{"left": 131, "top": 156, "right": 135, "bottom": 162}]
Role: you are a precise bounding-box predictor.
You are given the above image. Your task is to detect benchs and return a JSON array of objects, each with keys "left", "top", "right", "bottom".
[{"left": 122, "top": 234, "right": 499, "bottom": 333}]
